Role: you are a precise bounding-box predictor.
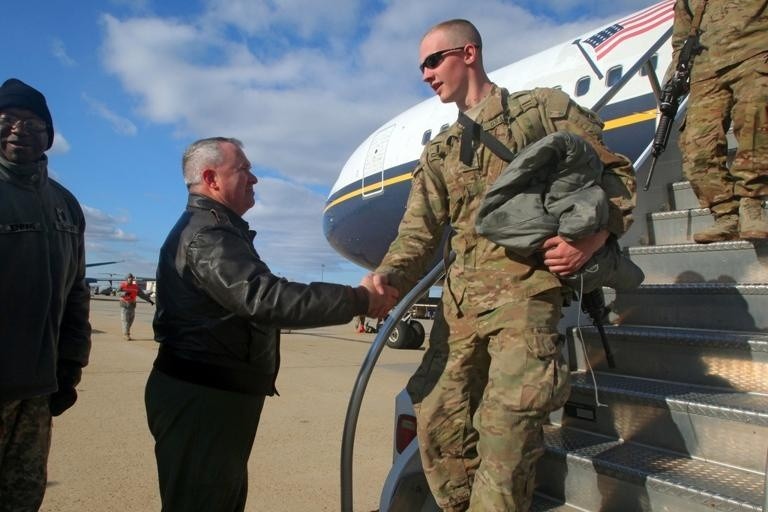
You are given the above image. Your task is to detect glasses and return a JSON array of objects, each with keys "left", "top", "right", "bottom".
[
  {"left": 1, "top": 112, "right": 49, "bottom": 132},
  {"left": 420, "top": 44, "right": 478, "bottom": 74}
]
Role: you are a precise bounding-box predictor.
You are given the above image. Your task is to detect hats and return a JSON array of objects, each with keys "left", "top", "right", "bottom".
[{"left": 0, "top": 78, "right": 53, "bottom": 146}]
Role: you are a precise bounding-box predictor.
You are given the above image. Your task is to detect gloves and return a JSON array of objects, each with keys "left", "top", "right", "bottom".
[{"left": 49, "top": 386, "right": 77, "bottom": 416}]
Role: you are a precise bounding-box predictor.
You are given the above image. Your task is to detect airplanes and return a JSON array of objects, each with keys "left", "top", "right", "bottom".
[
  {"left": 85, "top": 260, "right": 156, "bottom": 297},
  {"left": 321, "top": 0, "right": 736, "bottom": 349}
]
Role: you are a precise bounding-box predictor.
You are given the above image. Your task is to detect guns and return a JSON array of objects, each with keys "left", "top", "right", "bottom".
[
  {"left": 642, "top": 33, "right": 698, "bottom": 191},
  {"left": 581, "top": 289, "right": 619, "bottom": 369}
]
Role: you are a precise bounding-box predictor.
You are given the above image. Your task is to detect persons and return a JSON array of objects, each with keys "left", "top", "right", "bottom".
[
  {"left": 363, "top": 17, "right": 636, "bottom": 512},
  {"left": 1, "top": 79, "right": 94, "bottom": 510},
  {"left": 667, "top": 2, "right": 768, "bottom": 244},
  {"left": 116, "top": 272, "right": 155, "bottom": 340},
  {"left": 144, "top": 135, "right": 399, "bottom": 512}
]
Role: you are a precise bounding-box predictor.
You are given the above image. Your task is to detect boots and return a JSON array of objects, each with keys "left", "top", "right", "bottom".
[
  {"left": 124, "top": 333, "right": 131, "bottom": 341},
  {"left": 738, "top": 199, "right": 768, "bottom": 242},
  {"left": 694, "top": 212, "right": 739, "bottom": 243}
]
